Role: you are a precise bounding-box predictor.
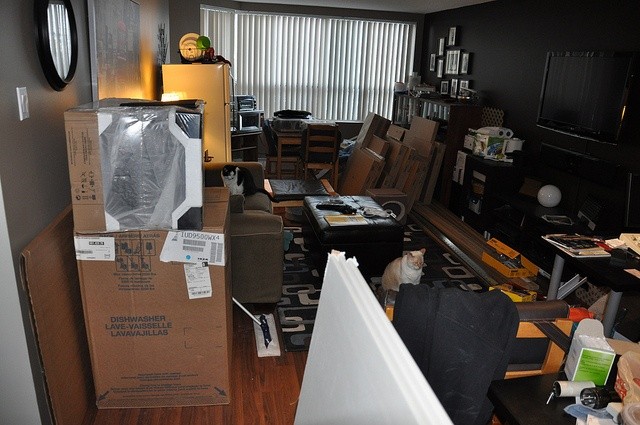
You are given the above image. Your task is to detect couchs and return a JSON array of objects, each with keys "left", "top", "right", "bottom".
[{"left": 202, "top": 162, "right": 294, "bottom": 303}]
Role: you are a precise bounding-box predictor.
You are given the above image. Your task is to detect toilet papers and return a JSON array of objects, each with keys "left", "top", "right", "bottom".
[{"left": 482, "top": 126, "right": 514, "bottom": 139}]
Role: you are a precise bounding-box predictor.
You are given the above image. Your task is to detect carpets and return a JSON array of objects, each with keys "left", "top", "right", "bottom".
[{"left": 274, "top": 206, "right": 487, "bottom": 351}]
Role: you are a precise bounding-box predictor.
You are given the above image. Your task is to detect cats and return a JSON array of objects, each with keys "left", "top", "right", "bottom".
[
  {"left": 221, "top": 164, "right": 280, "bottom": 202},
  {"left": 382, "top": 248, "right": 426, "bottom": 292}
]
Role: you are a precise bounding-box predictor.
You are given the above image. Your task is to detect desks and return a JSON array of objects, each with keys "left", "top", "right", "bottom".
[
  {"left": 301, "top": 196, "right": 405, "bottom": 276},
  {"left": 270, "top": 123, "right": 338, "bottom": 180},
  {"left": 264, "top": 178, "right": 338, "bottom": 215},
  {"left": 538, "top": 229, "right": 640, "bottom": 338},
  {"left": 488, "top": 366, "right": 626, "bottom": 425}
]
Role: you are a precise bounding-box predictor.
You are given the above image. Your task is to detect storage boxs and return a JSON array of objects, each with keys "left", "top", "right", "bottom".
[
  {"left": 387, "top": 136, "right": 418, "bottom": 191},
  {"left": 354, "top": 112, "right": 392, "bottom": 149},
  {"left": 65, "top": 98, "right": 203, "bottom": 235},
  {"left": 72, "top": 186, "right": 230, "bottom": 410},
  {"left": 410, "top": 116, "right": 438, "bottom": 143},
  {"left": 402, "top": 131, "right": 435, "bottom": 200},
  {"left": 421, "top": 141, "right": 446, "bottom": 205},
  {"left": 377, "top": 136, "right": 407, "bottom": 188},
  {"left": 387, "top": 123, "right": 408, "bottom": 141},
  {"left": 367, "top": 134, "right": 389, "bottom": 156},
  {"left": 404, "top": 158, "right": 425, "bottom": 214},
  {"left": 340, "top": 149, "right": 380, "bottom": 195},
  {"left": 361, "top": 148, "right": 385, "bottom": 188}
]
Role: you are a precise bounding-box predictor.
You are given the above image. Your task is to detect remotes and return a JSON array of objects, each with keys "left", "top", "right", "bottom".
[{"left": 315, "top": 203, "right": 358, "bottom": 214}]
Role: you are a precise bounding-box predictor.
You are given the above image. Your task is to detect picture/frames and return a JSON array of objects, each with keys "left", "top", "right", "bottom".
[
  {"left": 88, "top": 0, "right": 140, "bottom": 106},
  {"left": 450, "top": 78, "right": 458, "bottom": 98},
  {"left": 444, "top": 49, "right": 461, "bottom": 75},
  {"left": 437, "top": 59, "right": 444, "bottom": 78},
  {"left": 440, "top": 79, "right": 449, "bottom": 94},
  {"left": 429, "top": 53, "right": 437, "bottom": 72},
  {"left": 447, "top": 26, "right": 458, "bottom": 46},
  {"left": 438, "top": 37, "right": 446, "bottom": 56},
  {"left": 458, "top": 52, "right": 471, "bottom": 74},
  {"left": 460, "top": 80, "right": 469, "bottom": 95}
]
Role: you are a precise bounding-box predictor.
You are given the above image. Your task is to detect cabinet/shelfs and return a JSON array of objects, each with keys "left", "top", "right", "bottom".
[
  {"left": 391, "top": 90, "right": 482, "bottom": 141},
  {"left": 448, "top": 150, "right": 519, "bottom": 226}
]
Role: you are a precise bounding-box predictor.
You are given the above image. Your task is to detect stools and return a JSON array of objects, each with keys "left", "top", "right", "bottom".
[{"left": 365, "top": 188, "right": 408, "bottom": 227}]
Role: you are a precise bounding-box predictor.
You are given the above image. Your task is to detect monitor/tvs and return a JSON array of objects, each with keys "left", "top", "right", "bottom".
[{"left": 533, "top": 45, "right": 640, "bottom": 146}]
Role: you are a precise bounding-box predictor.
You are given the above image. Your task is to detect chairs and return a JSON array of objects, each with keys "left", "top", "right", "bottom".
[
  {"left": 259, "top": 113, "right": 300, "bottom": 180},
  {"left": 301, "top": 123, "right": 339, "bottom": 193},
  {"left": 384, "top": 290, "right": 573, "bottom": 381}
]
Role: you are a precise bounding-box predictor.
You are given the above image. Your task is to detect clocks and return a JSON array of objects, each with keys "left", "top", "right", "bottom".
[{"left": 34, "top": 0, "right": 78, "bottom": 92}]
